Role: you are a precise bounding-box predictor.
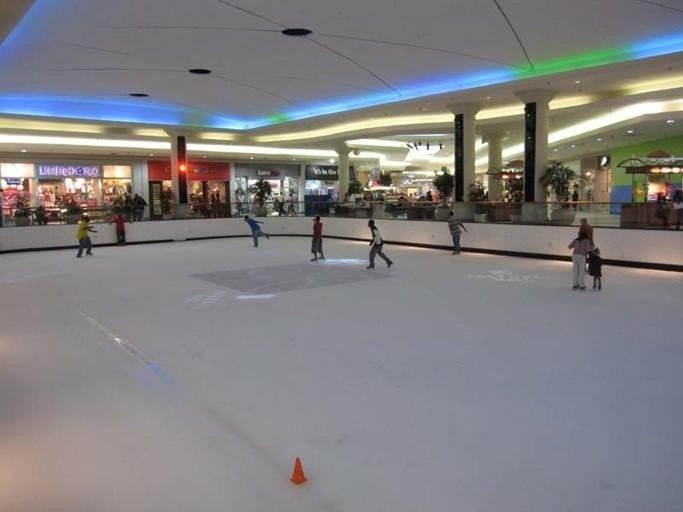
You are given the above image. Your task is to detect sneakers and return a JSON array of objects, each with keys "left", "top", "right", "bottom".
[
  {"left": 253, "top": 245, "right": 258, "bottom": 247},
  {"left": 365, "top": 265, "right": 375, "bottom": 269},
  {"left": 318, "top": 257, "right": 325, "bottom": 260},
  {"left": 310, "top": 258, "right": 318, "bottom": 261},
  {"left": 85, "top": 251, "right": 93, "bottom": 254},
  {"left": 265, "top": 232, "right": 268, "bottom": 238},
  {"left": 76, "top": 255, "right": 82, "bottom": 257},
  {"left": 579, "top": 286, "right": 586, "bottom": 289},
  {"left": 572, "top": 286, "right": 579, "bottom": 289},
  {"left": 387, "top": 261, "right": 393, "bottom": 268}
]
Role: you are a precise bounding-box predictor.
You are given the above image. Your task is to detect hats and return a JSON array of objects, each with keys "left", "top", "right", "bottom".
[{"left": 244, "top": 216, "right": 248, "bottom": 220}]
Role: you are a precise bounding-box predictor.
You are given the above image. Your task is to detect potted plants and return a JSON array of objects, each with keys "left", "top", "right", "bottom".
[
  {"left": 158, "top": 191, "right": 173, "bottom": 220},
  {"left": 14, "top": 203, "right": 83, "bottom": 226},
  {"left": 433, "top": 172, "right": 454, "bottom": 221},
  {"left": 247, "top": 177, "right": 273, "bottom": 216},
  {"left": 535, "top": 160, "right": 578, "bottom": 225},
  {"left": 467, "top": 189, "right": 487, "bottom": 222}
]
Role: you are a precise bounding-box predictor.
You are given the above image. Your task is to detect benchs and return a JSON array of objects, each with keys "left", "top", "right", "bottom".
[{"left": 405, "top": 205, "right": 434, "bottom": 220}]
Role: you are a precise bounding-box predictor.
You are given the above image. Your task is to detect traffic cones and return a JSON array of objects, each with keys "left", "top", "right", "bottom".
[{"left": 289, "top": 455, "right": 307, "bottom": 485}]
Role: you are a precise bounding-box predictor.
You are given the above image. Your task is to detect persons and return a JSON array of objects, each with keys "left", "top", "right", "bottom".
[
  {"left": 109, "top": 213, "right": 125, "bottom": 244},
  {"left": 652, "top": 191, "right": 670, "bottom": 230},
  {"left": 566, "top": 233, "right": 594, "bottom": 291},
  {"left": 447, "top": 210, "right": 468, "bottom": 254},
  {"left": 671, "top": 188, "right": 682, "bottom": 230},
  {"left": 577, "top": 217, "right": 593, "bottom": 250},
  {"left": 76, "top": 216, "right": 97, "bottom": 258},
  {"left": 243, "top": 215, "right": 269, "bottom": 249},
  {"left": 310, "top": 216, "right": 324, "bottom": 261},
  {"left": 364, "top": 218, "right": 393, "bottom": 269},
  {"left": 588, "top": 248, "right": 609, "bottom": 290}
]
